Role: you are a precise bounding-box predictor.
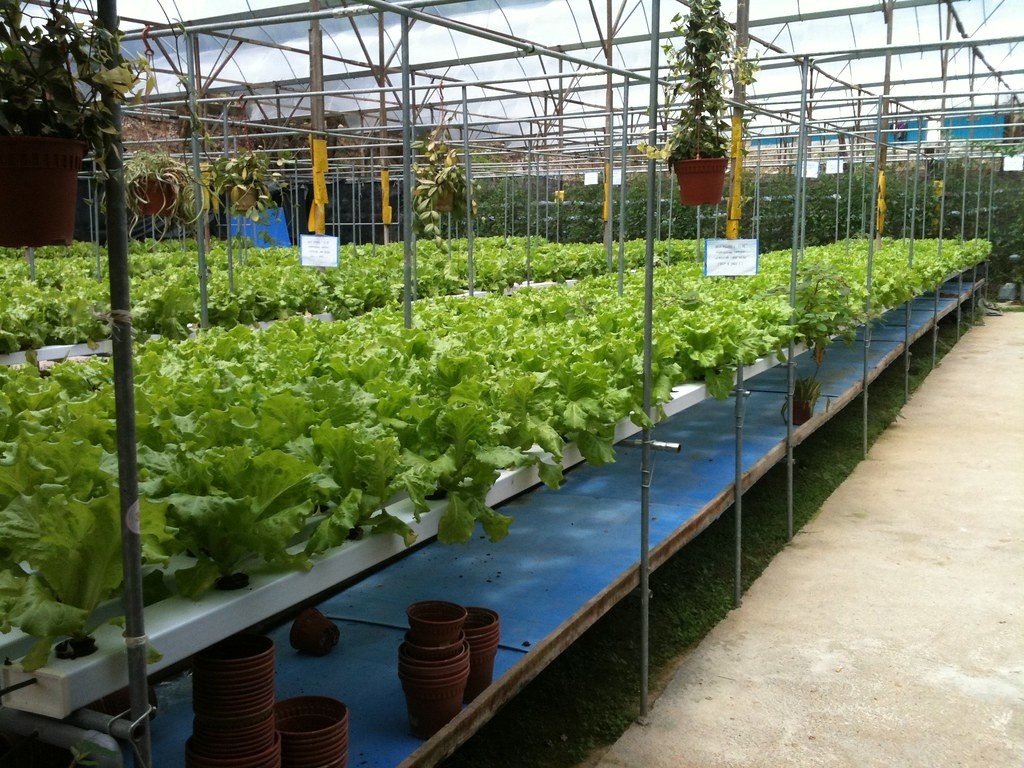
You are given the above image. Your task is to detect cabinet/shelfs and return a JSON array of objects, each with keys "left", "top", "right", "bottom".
[{"left": 0, "top": 230, "right": 991, "bottom": 767}]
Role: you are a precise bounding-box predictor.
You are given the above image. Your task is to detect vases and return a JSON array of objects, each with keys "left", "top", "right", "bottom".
[
  {"left": 396, "top": 600, "right": 501, "bottom": 737},
  {"left": 183, "top": 635, "right": 350, "bottom": 768},
  {"left": 289, "top": 608, "right": 341, "bottom": 654}
]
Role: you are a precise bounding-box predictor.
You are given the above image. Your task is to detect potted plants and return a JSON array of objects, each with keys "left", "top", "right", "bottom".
[
  {"left": 782, "top": 366, "right": 823, "bottom": 426},
  {"left": 635, "top": 0, "right": 758, "bottom": 207},
  {"left": 410, "top": 117, "right": 482, "bottom": 254},
  {"left": 207, "top": 148, "right": 294, "bottom": 249},
  {"left": 82, "top": 143, "right": 199, "bottom": 250},
  {"left": 1, "top": 0, "right": 158, "bottom": 249}
]
